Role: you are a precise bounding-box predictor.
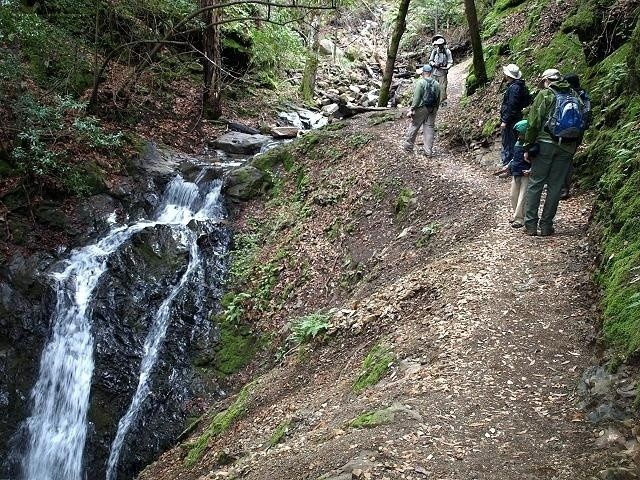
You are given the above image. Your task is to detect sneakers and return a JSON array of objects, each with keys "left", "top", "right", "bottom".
[{"left": 509, "top": 213, "right": 554, "bottom": 236}]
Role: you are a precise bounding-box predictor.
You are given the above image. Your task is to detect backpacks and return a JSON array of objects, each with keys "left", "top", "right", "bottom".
[
  {"left": 544, "top": 87, "right": 585, "bottom": 145},
  {"left": 422, "top": 78, "right": 436, "bottom": 113}
]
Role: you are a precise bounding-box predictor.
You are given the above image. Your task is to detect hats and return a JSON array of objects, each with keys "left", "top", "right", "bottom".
[
  {"left": 423, "top": 65, "right": 432, "bottom": 71},
  {"left": 538, "top": 69, "right": 562, "bottom": 85},
  {"left": 432, "top": 38, "right": 444, "bottom": 45},
  {"left": 503, "top": 64, "right": 522, "bottom": 80}
]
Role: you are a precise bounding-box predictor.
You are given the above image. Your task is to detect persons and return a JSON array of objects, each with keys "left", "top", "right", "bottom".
[
  {"left": 503, "top": 119, "right": 530, "bottom": 228},
  {"left": 522, "top": 68, "right": 585, "bottom": 236},
  {"left": 559, "top": 72, "right": 593, "bottom": 202},
  {"left": 497, "top": 63, "right": 531, "bottom": 168},
  {"left": 430, "top": 38, "right": 455, "bottom": 105},
  {"left": 402, "top": 64, "right": 441, "bottom": 160}
]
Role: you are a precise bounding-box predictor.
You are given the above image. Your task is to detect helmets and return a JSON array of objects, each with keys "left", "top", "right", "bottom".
[{"left": 513, "top": 120, "right": 527, "bottom": 133}]
